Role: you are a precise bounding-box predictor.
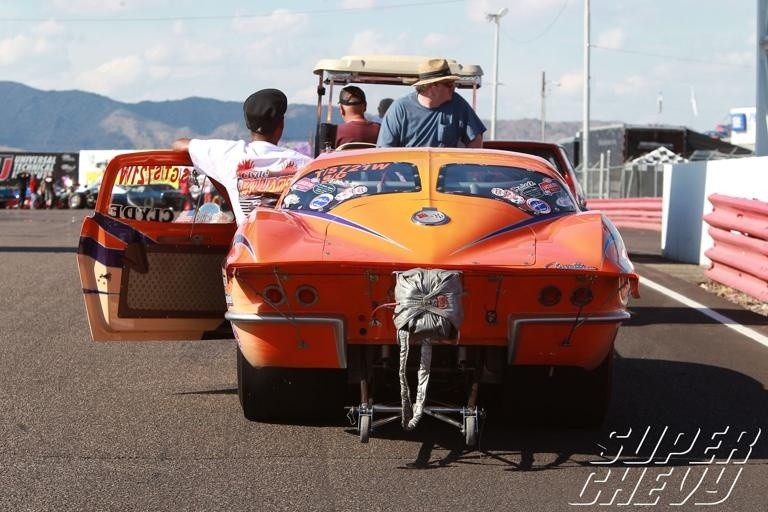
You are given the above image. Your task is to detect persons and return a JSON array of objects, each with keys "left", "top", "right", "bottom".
[
  {"left": 178, "top": 167, "right": 212, "bottom": 210},
  {"left": 172, "top": 88, "right": 315, "bottom": 227},
  {"left": 15, "top": 170, "right": 56, "bottom": 209},
  {"left": 319, "top": 85, "right": 381, "bottom": 152},
  {"left": 376, "top": 59, "right": 487, "bottom": 149}
]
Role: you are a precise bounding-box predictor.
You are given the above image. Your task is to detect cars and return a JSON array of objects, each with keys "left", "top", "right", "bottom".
[
  {"left": 128, "top": 183, "right": 186, "bottom": 212},
  {"left": 67, "top": 183, "right": 127, "bottom": 212}
]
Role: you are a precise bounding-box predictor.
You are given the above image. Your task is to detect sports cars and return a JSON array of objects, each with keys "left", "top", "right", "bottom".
[
  {"left": 77, "top": 144, "right": 642, "bottom": 432},
  {"left": 482, "top": 139, "right": 588, "bottom": 210}
]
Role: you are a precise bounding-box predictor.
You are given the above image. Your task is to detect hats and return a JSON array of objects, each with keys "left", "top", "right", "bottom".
[
  {"left": 337, "top": 85, "right": 367, "bottom": 107},
  {"left": 412, "top": 58, "right": 462, "bottom": 88},
  {"left": 242, "top": 88, "right": 288, "bottom": 135}
]
